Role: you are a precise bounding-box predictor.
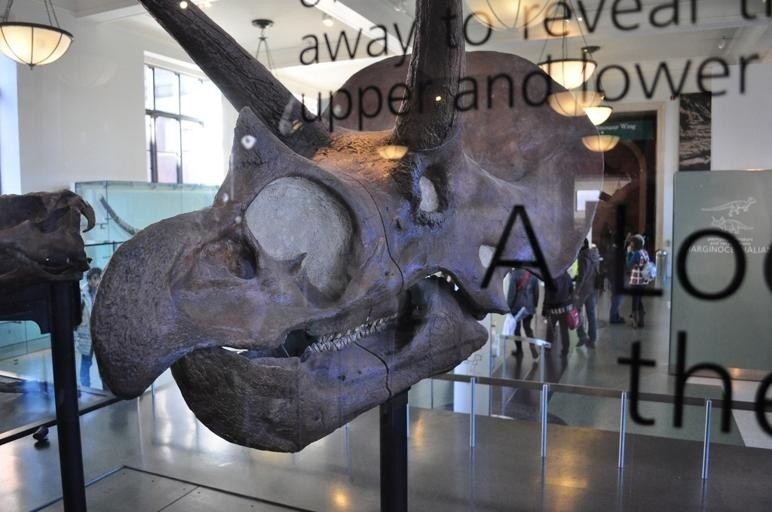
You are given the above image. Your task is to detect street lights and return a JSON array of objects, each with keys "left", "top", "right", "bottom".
[
  {"left": 566, "top": 306, "right": 582, "bottom": 329},
  {"left": 596, "top": 273, "right": 605, "bottom": 291}
]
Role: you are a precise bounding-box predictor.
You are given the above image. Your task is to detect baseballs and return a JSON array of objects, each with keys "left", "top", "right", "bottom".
[{"left": 654, "top": 247, "right": 667, "bottom": 289}]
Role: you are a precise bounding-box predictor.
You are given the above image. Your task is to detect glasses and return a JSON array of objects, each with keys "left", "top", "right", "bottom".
[
  {"left": 464, "top": 0, "right": 622, "bottom": 153},
  {"left": 465, "top": 1, "right": 620, "bottom": 127},
  {"left": 1, "top": 0, "right": 75, "bottom": 71}
]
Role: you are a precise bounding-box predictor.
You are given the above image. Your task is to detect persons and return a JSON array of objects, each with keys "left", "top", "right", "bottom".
[
  {"left": 76, "top": 267, "right": 101, "bottom": 386},
  {"left": 626, "top": 234, "right": 650, "bottom": 329},
  {"left": 592, "top": 223, "right": 653, "bottom": 317},
  {"left": 608, "top": 233, "right": 626, "bottom": 324},
  {"left": 507, "top": 266, "right": 538, "bottom": 360},
  {"left": 540, "top": 270, "right": 573, "bottom": 357}
]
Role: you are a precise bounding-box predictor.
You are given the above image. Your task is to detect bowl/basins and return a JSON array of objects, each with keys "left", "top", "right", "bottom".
[{"left": 572, "top": 238, "right": 598, "bottom": 350}]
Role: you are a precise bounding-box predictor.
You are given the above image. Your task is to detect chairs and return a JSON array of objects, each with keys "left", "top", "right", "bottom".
[
  {"left": 609, "top": 312, "right": 647, "bottom": 329},
  {"left": 511, "top": 337, "right": 596, "bottom": 360}
]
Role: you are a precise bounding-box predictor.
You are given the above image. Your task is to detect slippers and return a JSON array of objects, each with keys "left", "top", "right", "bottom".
[{"left": 640, "top": 251, "right": 657, "bottom": 282}]
